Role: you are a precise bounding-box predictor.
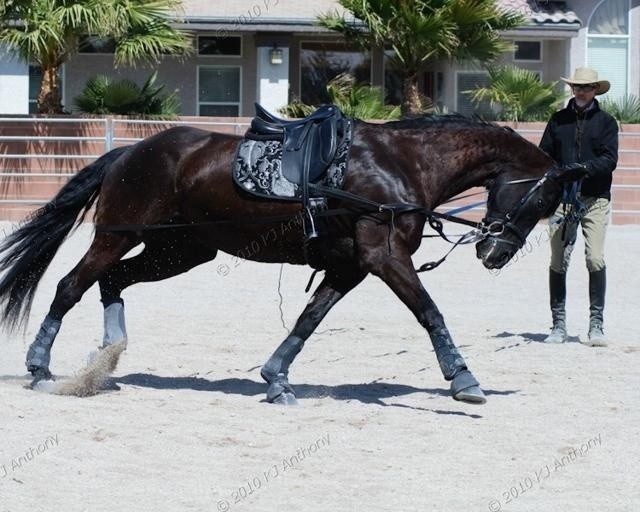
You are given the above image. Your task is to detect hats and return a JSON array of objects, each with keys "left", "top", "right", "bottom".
[{"left": 559, "top": 67, "right": 611, "bottom": 95}]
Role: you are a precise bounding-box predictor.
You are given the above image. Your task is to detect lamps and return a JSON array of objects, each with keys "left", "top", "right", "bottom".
[{"left": 269, "top": 49, "right": 283, "bottom": 65}]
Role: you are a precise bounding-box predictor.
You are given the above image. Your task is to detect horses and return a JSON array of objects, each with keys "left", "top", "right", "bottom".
[{"left": 1, "top": 107, "right": 588, "bottom": 409}]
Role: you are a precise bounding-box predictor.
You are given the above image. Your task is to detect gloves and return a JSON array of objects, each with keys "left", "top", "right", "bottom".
[{"left": 566, "top": 162, "right": 584, "bottom": 183}]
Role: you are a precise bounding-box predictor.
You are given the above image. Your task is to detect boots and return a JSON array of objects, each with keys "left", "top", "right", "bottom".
[
  {"left": 543, "top": 266, "right": 567, "bottom": 344},
  {"left": 587, "top": 265, "right": 609, "bottom": 347}
]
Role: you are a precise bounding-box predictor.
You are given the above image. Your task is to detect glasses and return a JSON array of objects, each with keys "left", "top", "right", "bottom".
[{"left": 573, "top": 85, "right": 598, "bottom": 92}]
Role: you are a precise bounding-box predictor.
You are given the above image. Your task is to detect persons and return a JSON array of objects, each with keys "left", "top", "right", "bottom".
[{"left": 538, "top": 68, "right": 619, "bottom": 347}]
